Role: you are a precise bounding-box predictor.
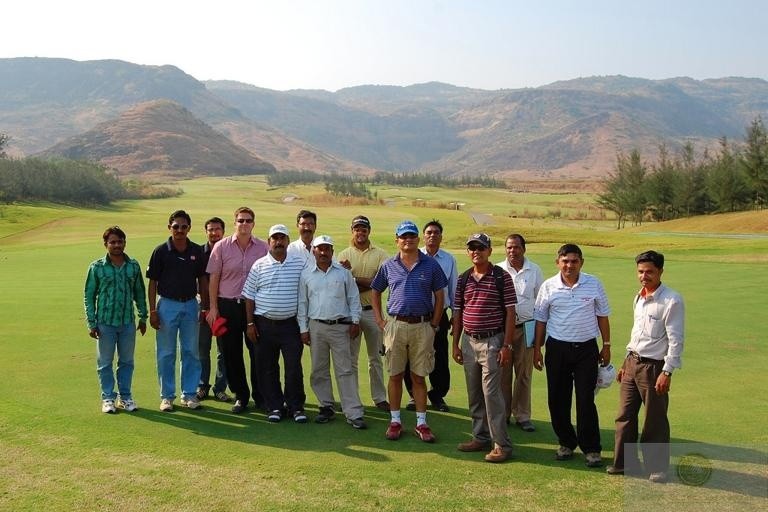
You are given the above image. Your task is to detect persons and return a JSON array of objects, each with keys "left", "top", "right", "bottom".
[
  {"left": 197, "top": 217, "right": 232, "bottom": 402},
  {"left": 604, "top": 250, "right": 685, "bottom": 483},
  {"left": 84, "top": 225, "right": 148, "bottom": 414},
  {"left": 287, "top": 210, "right": 335, "bottom": 270},
  {"left": 296, "top": 235, "right": 368, "bottom": 429},
  {"left": 453, "top": 233, "right": 518, "bottom": 462},
  {"left": 205, "top": 207, "right": 270, "bottom": 413},
  {"left": 403, "top": 219, "right": 458, "bottom": 412},
  {"left": 241, "top": 224, "right": 352, "bottom": 423},
  {"left": 370, "top": 221, "right": 448, "bottom": 443},
  {"left": 337, "top": 216, "right": 391, "bottom": 411},
  {"left": 493, "top": 234, "right": 544, "bottom": 432},
  {"left": 146, "top": 210, "right": 209, "bottom": 411},
  {"left": 533, "top": 244, "right": 611, "bottom": 466}
]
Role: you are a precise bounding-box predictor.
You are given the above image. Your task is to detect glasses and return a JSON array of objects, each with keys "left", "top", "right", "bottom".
[
  {"left": 299, "top": 222, "right": 316, "bottom": 226},
  {"left": 170, "top": 223, "right": 189, "bottom": 230},
  {"left": 236, "top": 218, "right": 254, "bottom": 224}
]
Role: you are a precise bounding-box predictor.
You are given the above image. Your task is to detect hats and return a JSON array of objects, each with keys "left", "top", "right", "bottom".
[
  {"left": 268, "top": 224, "right": 289, "bottom": 237},
  {"left": 351, "top": 215, "right": 371, "bottom": 229},
  {"left": 594, "top": 359, "right": 615, "bottom": 396},
  {"left": 314, "top": 235, "right": 334, "bottom": 247},
  {"left": 466, "top": 232, "right": 491, "bottom": 248},
  {"left": 395, "top": 220, "right": 419, "bottom": 235}
]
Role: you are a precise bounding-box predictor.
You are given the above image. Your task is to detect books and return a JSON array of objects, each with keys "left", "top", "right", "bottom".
[{"left": 523, "top": 320, "right": 546, "bottom": 349}]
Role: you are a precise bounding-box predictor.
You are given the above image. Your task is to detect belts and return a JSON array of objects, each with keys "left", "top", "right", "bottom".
[
  {"left": 362, "top": 305, "right": 372, "bottom": 311},
  {"left": 390, "top": 313, "right": 432, "bottom": 324},
  {"left": 630, "top": 352, "right": 664, "bottom": 362},
  {"left": 218, "top": 297, "right": 246, "bottom": 303},
  {"left": 464, "top": 328, "right": 505, "bottom": 339},
  {"left": 313, "top": 317, "right": 353, "bottom": 325},
  {"left": 168, "top": 294, "right": 195, "bottom": 302}
]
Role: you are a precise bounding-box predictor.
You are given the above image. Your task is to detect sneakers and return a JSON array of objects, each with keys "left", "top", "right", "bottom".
[
  {"left": 377, "top": 400, "right": 390, "bottom": 410},
  {"left": 293, "top": 410, "right": 308, "bottom": 422},
  {"left": 415, "top": 423, "right": 435, "bottom": 442},
  {"left": 385, "top": 422, "right": 404, "bottom": 441},
  {"left": 117, "top": 396, "right": 138, "bottom": 412},
  {"left": 584, "top": 452, "right": 604, "bottom": 468},
  {"left": 347, "top": 416, "right": 368, "bottom": 429},
  {"left": 214, "top": 391, "right": 231, "bottom": 401},
  {"left": 269, "top": 409, "right": 282, "bottom": 422},
  {"left": 315, "top": 406, "right": 336, "bottom": 423},
  {"left": 516, "top": 418, "right": 535, "bottom": 432},
  {"left": 160, "top": 398, "right": 173, "bottom": 411},
  {"left": 180, "top": 396, "right": 200, "bottom": 410},
  {"left": 196, "top": 386, "right": 209, "bottom": 399},
  {"left": 607, "top": 460, "right": 642, "bottom": 476},
  {"left": 102, "top": 399, "right": 116, "bottom": 413},
  {"left": 555, "top": 446, "right": 573, "bottom": 460},
  {"left": 650, "top": 470, "right": 666, "bottom": 482}
]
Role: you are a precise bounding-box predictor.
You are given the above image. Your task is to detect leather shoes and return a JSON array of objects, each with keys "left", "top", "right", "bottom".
[
  {"left": 457, "top": 438, "right": 492, "bottom": 451},
  {"left": 428, "top": 389, "right": 448, "bottom": 412},
  {"left": 484, "top": 444, "right": 513, "bottom": 462},
  {"left": 232, "top": 399, "right": 247, "bottom": 413},
  {"left": 406, "top": 398, "right": 416, "bottom": 410}
]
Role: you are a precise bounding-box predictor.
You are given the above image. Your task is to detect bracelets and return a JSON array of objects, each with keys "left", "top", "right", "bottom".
[
  {"left": 603, "top": 341, "right": 612, "bottom": 346},
  {"left": 149, "top": 309, "right": 158, "bottom": 313},
  {"left": 245, "top": 322, "right": 256, "bottom": 327}
]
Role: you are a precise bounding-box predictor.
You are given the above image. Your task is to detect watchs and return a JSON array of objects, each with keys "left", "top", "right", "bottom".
[
  {"left": 662, "top": 370, "right": 672, "bottom": 376},
  {"left": 353, "top": 320, "right": 360, "bottom": 324},
  {"left": 430, "top": 323, "right": 440, "bottom": 333},
  {"left": 503, "top": 343, "right": 516, "bottom": 352}
]
